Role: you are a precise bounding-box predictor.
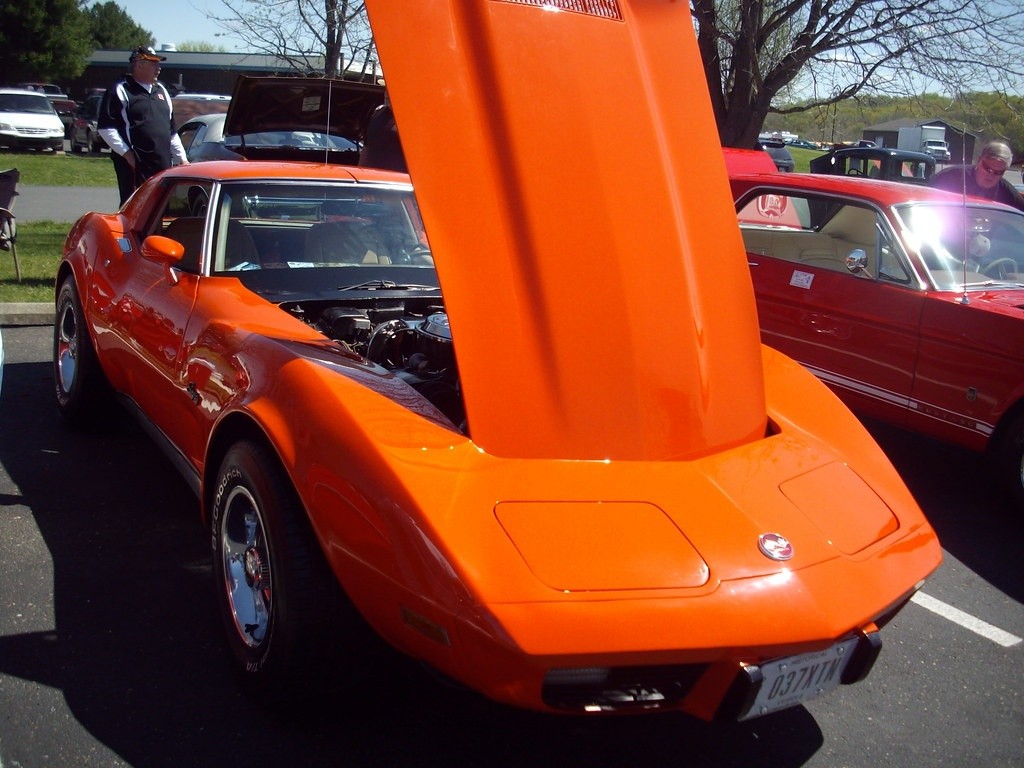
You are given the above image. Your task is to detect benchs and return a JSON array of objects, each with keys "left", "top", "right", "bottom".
[{"left": 740, "top": 204, "right": 890, "bottom": 279}]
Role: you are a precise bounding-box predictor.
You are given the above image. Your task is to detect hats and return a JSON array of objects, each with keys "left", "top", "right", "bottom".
[{"left": 129, "top": 45, "right": 167, "bottom": 61}]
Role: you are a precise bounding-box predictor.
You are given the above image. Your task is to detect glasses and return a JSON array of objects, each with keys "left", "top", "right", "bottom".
[{"left": 981, "top": 155, "right": 1006, "bottom": 176}]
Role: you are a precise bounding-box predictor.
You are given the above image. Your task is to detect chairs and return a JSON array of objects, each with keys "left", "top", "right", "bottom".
[
  {"left": 301, "top": 218, "right": 392, "bottom": 265},
  {"left": 0, "top": 166, "right": 22, "bottom": 283},
  {"left": 162, "top": 215, "right": 262, "bottom": 274}
]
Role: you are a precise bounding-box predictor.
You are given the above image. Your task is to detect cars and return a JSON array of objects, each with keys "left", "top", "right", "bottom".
[
  {"left": 1, "top": 74, "right": 437, "bottom": 268},
  {"left": 757, "top": 133, "right": 1023, "bottom": 231}
]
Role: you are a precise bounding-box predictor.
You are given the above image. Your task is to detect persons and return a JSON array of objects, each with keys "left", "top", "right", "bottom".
[
  {"left": 358, "top": 88, "right": 409, "bottom": 175},
  {"left": 928, "top": 142, "right": 1024, "bottom": 212},
  {"left": 97, "top": 45, "right": 190, "bottom": 210}
]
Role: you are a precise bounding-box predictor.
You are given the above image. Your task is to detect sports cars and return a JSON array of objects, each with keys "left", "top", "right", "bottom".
[
  {"left": 51, "top": 2, "right": 946, "bottom": 728},
  {"left": 719, "top": 144, "right": 1024, "bottom": 512}
]
